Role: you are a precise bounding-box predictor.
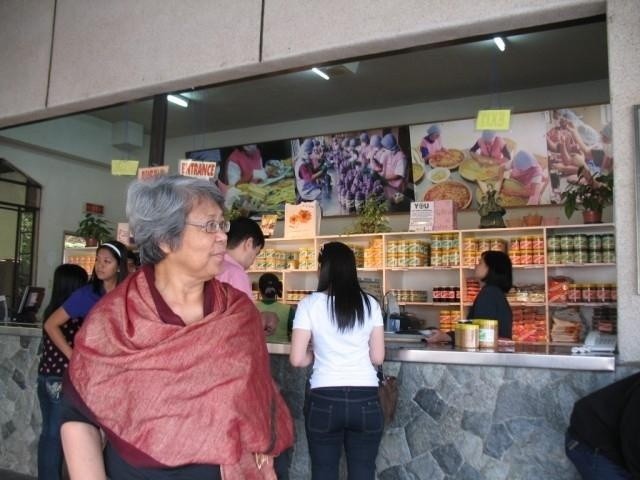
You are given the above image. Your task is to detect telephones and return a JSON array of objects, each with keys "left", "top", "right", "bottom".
[{"left": 585, "top": 331, "right": 617, "bottom": 352}]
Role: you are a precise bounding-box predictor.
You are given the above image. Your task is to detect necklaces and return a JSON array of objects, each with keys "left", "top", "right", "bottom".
[{"left": 104, "top": 280, "right": 116, "bottom": 290}]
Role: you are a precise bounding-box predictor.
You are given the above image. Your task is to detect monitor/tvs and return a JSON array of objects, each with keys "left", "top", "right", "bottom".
[{"left": 17, "top": 286, "right": 45, "bottom": 323}]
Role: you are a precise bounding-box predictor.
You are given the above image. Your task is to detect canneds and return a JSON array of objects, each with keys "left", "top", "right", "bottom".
[
  {"left": 285, "top": 289, "right": 317, "bottom": 301},
  {"left": 439, "top": 309, "right": 498, "bottom": 349},
  {"left": 568, "top": 283, "right": 617, "bottom": 303},
  {"left": 349, "top": 233, "right": 615, "bottom": 267},
  {"left": 255, "top": 247, "right": 315, "bottom": 269},
  {"left": 388, "top": 288, "right": 428, "bottom": 302},
  {"left": 432, "top": 285, "right": 461, "bottom": 302},
  {"left": 252, "top": 280, "right": 283, "bottom": 301}
]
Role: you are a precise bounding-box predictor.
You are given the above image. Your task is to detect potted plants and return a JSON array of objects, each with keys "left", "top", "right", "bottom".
[
  {"left": 75, "top": 210, "right": 114, "bottom": 248},
  {"left": 344, "top": 190, "right": 393, "bottom": 234},
  {"left": 557, "top": 168, "right": 612, "bottom": 222}
]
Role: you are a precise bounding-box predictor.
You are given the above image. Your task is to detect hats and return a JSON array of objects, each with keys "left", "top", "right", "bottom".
[
  {"left": 483, "top": 131, "right": 494, "bottom": 143},
  {"left": 426, "top": 125, "right": 442, "bottom": 135},
  {"left": 300, "top": 132, "right": 397, "bottom": 159},
  {"left": 190, "top": 150, "right": 221, "bottom": 162},
  {"left": 515, "top": 151, "right": 533, "bottom": 170}
]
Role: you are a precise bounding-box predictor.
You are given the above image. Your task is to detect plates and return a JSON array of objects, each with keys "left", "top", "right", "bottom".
[{"left": 428, "top": 166, "right": 450, "bottom": 184}]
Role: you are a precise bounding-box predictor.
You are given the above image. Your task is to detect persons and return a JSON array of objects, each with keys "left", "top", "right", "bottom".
[
  {"left": 290, "top": 241, "right": 389, "bottom": 479},
  {"left": 495, "top": 150, "right": 545, "bottom": 199},
  {"left": 44, "top": 241, "right": 128, "bottom": 361},
  {"left": 253, "top": 273, "right": 297, "bottom": 343},
  {"left": 468, "top": 129, "right": 512, "bottom": 168},
  {"left": 295, "top": 132, "right": 409, "bottom": 195},
  {"left": 550, "top": 112, "right": 612, "bottom": 189},
  {"left": 420, "top": 123, "right": 449, "bottom": 160},
  {"left": 34, "top": 263, "right": 89, "bottom": 479},
  {"left": 211, "top": 216, "right": 267, "bottom": 303},
  {"left": 191, "top": 149, "right": 230, "bottom": 192},
  {"left": 226, "top": 144, "right": 266, "bottom": 185},
  {"left": 58, "top": 175, "right": 295, "bottom": 480},
  {"left": 425, "top": 249, "right": 513, "bottom": 345},
  {"left": 565, "top": 372, "right": 639, "bottom": 480}
]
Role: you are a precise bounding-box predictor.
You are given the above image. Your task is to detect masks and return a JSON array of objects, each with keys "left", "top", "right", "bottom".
[
  {"left": 552, "top": 118, "right": 560, "bottom": 126},
  {"left": 243, "top": 145, "right": 257, "bottom": 155},
  {"left": 603, "top": 144, "right": 612, "bottom": 158}
]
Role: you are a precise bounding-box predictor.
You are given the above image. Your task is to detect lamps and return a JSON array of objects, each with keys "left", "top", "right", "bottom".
[
  {"left": 312, "top": 68, "right": 331, "bottom": 82},
  {"left": 494, "top": 35, "right": 509, "bottom": 53},
  {"left": 165, "top": 93, "right": 191, "bottom": 108}
]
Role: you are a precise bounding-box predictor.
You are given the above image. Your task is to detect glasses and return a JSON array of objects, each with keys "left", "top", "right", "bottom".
[{"left": 187, "top": 220, "right": 231, "bottom": 233}]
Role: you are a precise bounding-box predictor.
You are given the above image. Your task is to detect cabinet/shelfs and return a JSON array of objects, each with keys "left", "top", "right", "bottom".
[
  {"left": 460, "top": 228, "right": 547, "bottom": 354},
  {"left": 385, "top": 230, "right": 460, "bottom": 332},
  {"left": 248, "top": 236, "right": 384, "bottom": 316},
  {"left": 544, "top": 223, "right": 615, "bottom": 356}
]
